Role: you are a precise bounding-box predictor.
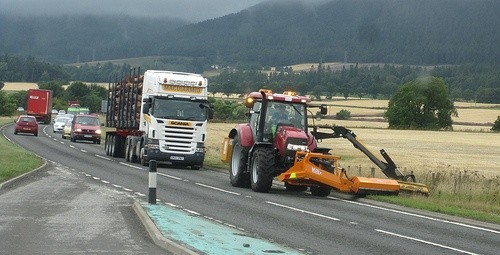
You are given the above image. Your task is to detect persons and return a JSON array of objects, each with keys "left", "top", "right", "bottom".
[{"left": 271, "top": 105, "right": 288, "bottom": 124}]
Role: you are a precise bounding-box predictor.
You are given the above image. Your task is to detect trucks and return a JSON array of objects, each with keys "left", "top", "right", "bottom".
[{"left": 27, "top": 89, "right": 53, "bottom": 124}]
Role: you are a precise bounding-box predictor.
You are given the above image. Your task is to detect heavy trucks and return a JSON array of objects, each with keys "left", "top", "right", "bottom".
[{"left": 102, "top": 69, "right": 216, "bottom": 170}]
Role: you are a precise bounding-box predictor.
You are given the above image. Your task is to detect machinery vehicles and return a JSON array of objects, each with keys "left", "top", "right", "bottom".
[{"left": 220, "top": 88, "right": 432, "bottom": 198}]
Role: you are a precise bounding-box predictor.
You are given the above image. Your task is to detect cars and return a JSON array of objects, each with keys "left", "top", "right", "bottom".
[
  {"left": 52, "top": 103, "right": 91, "bottom": 132},
  {"left": 70, "top": 113, "right": 104, "bottom": 144},
  {"left": 16, "top": 106, "right": 25, "bottom": 112},
  {"left": 61, "top": 119, "right": 73, "bottom": 139},
  {"left": 13, "top": 114, "right": 39, "bottom": 136}
]
